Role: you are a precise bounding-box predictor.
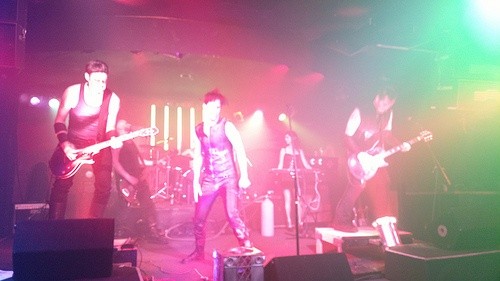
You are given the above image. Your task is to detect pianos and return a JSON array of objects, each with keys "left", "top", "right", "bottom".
[{"left": 268, "top": 166, "right": 332, "bottom": 188}]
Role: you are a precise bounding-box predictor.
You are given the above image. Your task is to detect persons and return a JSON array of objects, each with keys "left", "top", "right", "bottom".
[
  {"left": 48, "top": 59, "right": 124, "bottom": 221},
  {"left": 180, "top": 92, "right": 254, "bottom": 261},
  {"left": 345, "top": 86, "right": 413, "bottom": 236},
  {"left": 112, "top": 119, "right": 159, "bottom": 240},
  {"left": 277, "top": 129, "right": 313, "bottom": 229}
]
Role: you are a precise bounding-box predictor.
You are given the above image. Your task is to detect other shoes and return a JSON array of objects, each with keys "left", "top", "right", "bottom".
[{"left": 181, "top": 248, "right": 204, "bottom": 264}]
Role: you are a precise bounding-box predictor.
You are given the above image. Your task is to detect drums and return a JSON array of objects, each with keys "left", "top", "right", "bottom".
[{"left": 137, "top": 143, "right": 192, "bottom": 173}]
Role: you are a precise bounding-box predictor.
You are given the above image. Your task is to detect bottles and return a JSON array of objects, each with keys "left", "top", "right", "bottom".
[{"left": 261, "top": 196, "right": 274, "bottom": 236}]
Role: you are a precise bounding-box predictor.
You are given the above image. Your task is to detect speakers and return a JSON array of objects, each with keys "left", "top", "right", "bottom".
[
  {"left": 11, "top": 218, "right": 114, "bottom": 281},
  {"left": 384, "top": 191, "right": 500, "bottom": 281},
  {"left": 264, "top": 252, "right": 354, "bottom": 281}
]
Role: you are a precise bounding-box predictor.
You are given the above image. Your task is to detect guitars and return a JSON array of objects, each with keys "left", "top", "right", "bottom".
[
  {"left": 48, "top": 125, "right": 158, "bottom": 180},
  {"left": 118, "top": 157, "right": 162, "bottom": 209},
  {"left": 345, "top": 130, "right": 433, "bottom": 183}
]
